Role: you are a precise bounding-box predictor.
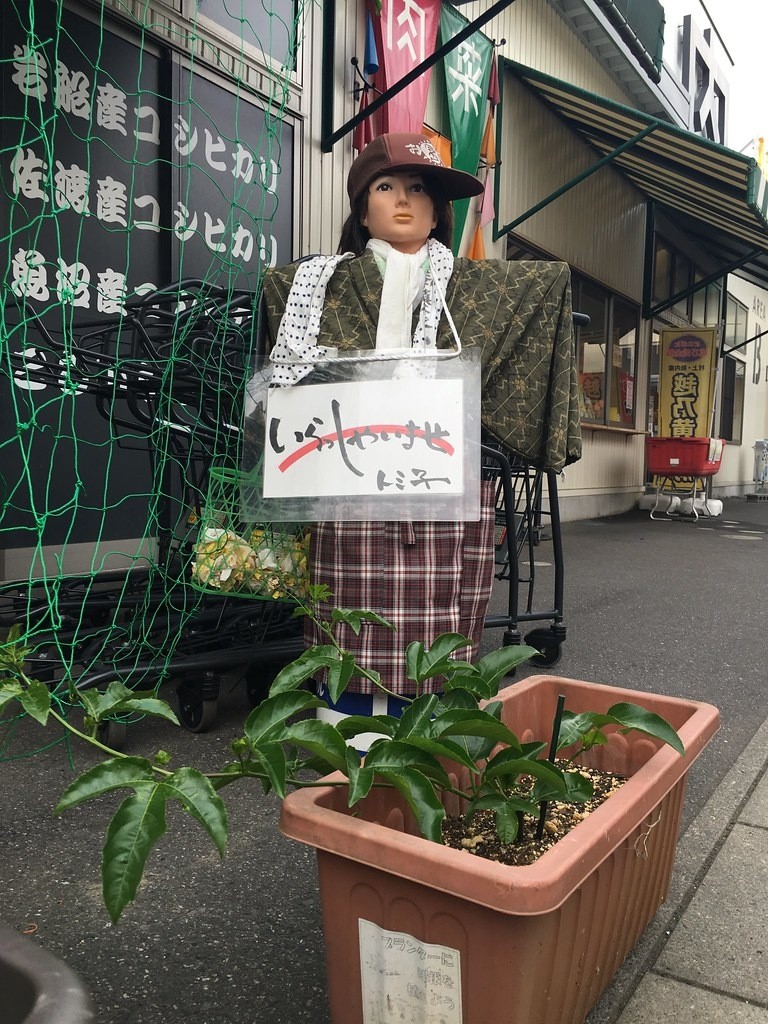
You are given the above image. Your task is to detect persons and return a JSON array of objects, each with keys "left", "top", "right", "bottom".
[{"left": 258, "top": 132, "right": 587, "bottom": 695}]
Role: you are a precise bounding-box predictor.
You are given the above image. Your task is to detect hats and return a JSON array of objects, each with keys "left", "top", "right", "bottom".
[{"left": 346, "top": 132, "right": 485, "bottom": 209}]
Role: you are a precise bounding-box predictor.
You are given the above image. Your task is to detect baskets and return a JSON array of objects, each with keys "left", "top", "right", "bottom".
[{"left": 191, "top": 447, "right": 311, "bottom": 602}]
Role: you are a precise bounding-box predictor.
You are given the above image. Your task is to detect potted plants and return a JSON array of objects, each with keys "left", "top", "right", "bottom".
[{"left": 276, "top": 675, "right": 721, "bottom": 1024}]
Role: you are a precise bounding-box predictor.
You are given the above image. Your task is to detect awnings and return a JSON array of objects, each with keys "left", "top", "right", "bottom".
[
  {"left": 644, "top": 199, "right": 767, "bottom": 324},
  {"left": 491, "top": 54, "right": 767, "bottom": 319}
]
